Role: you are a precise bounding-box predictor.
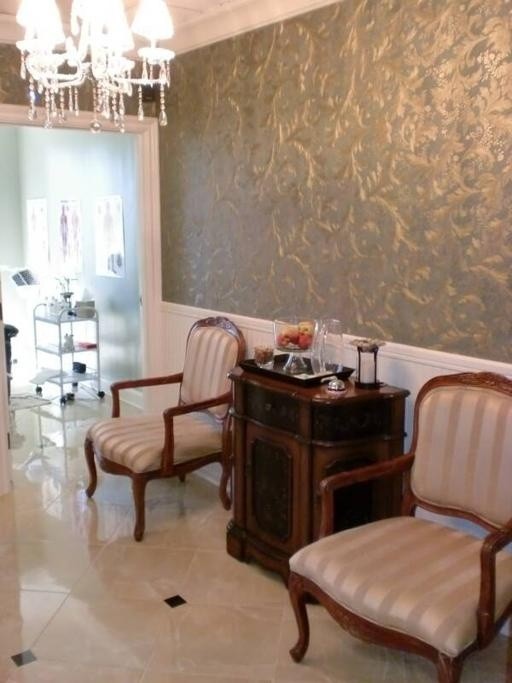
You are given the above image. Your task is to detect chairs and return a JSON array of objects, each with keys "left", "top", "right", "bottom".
[
  {"left": 283, "top": 369, "right": 512, "bottom": 683},
  {"left": 80, "top": 314, "right": 247, "bottom": 541}
]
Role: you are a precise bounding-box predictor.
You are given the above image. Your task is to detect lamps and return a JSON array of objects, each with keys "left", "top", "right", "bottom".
[{"left": 10, "top": 0, "right": 179, "bottom": 132}]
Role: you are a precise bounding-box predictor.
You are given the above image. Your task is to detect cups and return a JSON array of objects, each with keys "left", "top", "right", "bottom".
[{"left": 254, "top": 346, "right": 273, "bottom": 366}]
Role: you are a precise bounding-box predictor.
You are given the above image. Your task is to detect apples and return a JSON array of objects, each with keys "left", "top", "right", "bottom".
[
  {"left": 298, "top": 333, "right": 312, "bottom": 350},
  {"left": 276, "top": 335, "right": 289, "bottom": 347},
  {"left": 282, "top": 326, "right": 297, "bottom": 342},
  {"left": 297, "top": 321, "right": 314, "bottom": 337}
]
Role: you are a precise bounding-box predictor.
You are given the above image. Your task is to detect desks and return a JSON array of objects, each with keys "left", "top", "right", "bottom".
[{"left": 225, "top": 358, "right": 411, "bottom": 591}]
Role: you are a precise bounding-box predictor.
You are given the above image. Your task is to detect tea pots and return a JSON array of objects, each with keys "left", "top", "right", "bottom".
[{"left": 310, "top": 318, "right": 344, "bottom": 374}]
